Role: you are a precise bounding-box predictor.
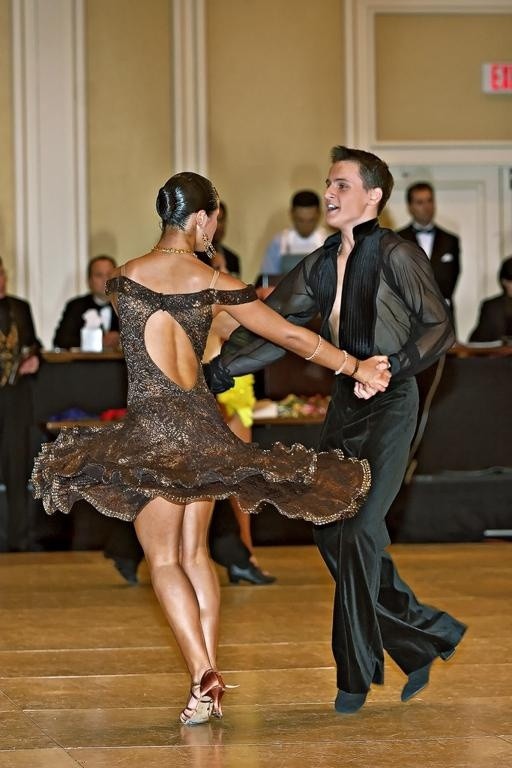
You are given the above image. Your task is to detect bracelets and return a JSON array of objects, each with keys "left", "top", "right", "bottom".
[
  {"left": 352, "top": 358, "right": 360, "bottom": 376},
  {"left": 334, "top": 350, "right": 349, "bottom": 376}
]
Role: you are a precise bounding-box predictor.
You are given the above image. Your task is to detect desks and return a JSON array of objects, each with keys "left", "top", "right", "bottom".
[{"left": 1, "top": 349, "right": 512, "bottom": 554}]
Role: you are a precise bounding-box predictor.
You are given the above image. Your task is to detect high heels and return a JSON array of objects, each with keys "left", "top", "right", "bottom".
[
  {"left": 334, "top": 687, "right": 367, "bottom": 712},
  {"left": 401, "top": 645, "right": 455, "bottom": 700},
  {"left": 180, "top": 670, "right": 226, "bottom": 724}
]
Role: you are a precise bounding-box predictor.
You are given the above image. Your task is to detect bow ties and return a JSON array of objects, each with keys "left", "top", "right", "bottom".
[{"left": 415, "top": 229, "right": 433, "bottom": 234}]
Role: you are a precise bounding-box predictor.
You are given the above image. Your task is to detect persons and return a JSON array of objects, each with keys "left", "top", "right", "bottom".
[
  {"left": 32, "top": 175, "right": 393, "bottom": 726},
  {"left": 470, "top": 256, "right": 512, "bottom": 344},
  {"left": 395, "top": 182, "right": 461, "bottom": 333},
  {"left": 2, "top": 205, "right": 279, "bottom": 584},
  {"left": 203, "top": 147, "right": 469, "bottom": 716},
  {"left": 256, "top": 188, "right": 334, "bottom": 299}
]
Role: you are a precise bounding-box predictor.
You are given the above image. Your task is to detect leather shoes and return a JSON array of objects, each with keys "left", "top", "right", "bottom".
[
  {"left": 228, "top": 562, "right": 275, "bottom": 584},
  {"left": 112, "top": 554, "right": 141, "bottom": 584}
]
